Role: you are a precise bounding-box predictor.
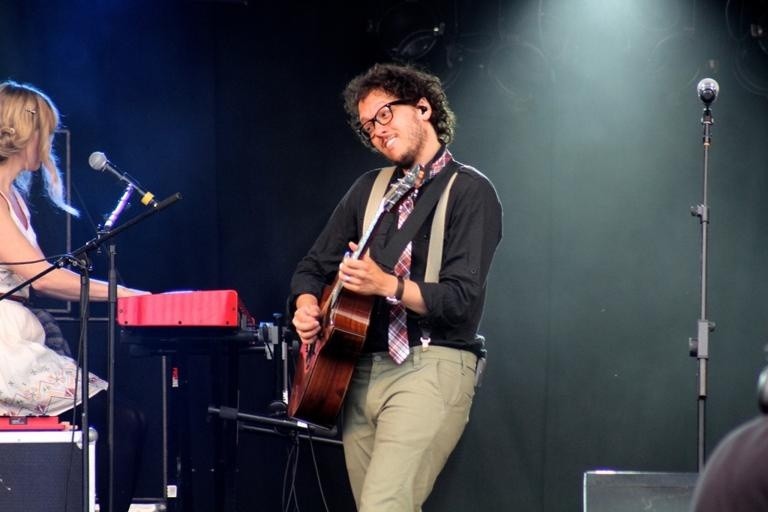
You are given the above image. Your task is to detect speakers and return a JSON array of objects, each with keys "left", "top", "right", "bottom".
[
  {"left": 583, "top": 470, "right": 701, "bottom": 512},
  {"left": 0, "top": 431, "right": 93, "bottom": 510}
]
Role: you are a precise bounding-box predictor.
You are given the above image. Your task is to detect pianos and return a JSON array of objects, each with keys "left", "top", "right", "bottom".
[{"left": 115, "top": 288, "right": 255, "bottom": 347}]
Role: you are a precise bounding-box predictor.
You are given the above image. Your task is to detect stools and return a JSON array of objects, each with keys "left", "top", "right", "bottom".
[{"left": 1, "top": 411, "right": 100, "bottom": 512}]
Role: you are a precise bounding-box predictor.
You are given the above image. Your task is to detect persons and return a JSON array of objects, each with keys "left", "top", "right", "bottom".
[
  {"left": 287, "top": 62, "right": 506, "bottom": 511},
  {"left": 0, "top": 78, "right": 160, "bottom": 511}
]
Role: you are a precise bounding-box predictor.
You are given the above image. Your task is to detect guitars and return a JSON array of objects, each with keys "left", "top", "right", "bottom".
[{"left": 287, "top": 164, "right": 427, "bottom": 430}]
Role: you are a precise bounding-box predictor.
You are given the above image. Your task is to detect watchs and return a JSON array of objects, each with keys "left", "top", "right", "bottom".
[{"left": 386, "top": 275, "right": 409, "bottom": 304}]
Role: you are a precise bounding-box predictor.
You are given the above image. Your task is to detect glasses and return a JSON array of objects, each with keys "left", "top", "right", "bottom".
[{"left": 358, "top": 96, "right": 416, "bottom": 141}]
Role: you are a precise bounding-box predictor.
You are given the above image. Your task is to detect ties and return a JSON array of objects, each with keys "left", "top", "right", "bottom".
[{"left": 384, "top": 146, "right": 456, "bottom": 365}]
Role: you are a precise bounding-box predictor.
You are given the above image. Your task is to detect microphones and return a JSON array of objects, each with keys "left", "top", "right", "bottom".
[
  {"left": 697, "top": 78, "right": 720, "bottom": 105},
  {"left": 88, "top": 151, "right": 155, "bottom": 204}
]
